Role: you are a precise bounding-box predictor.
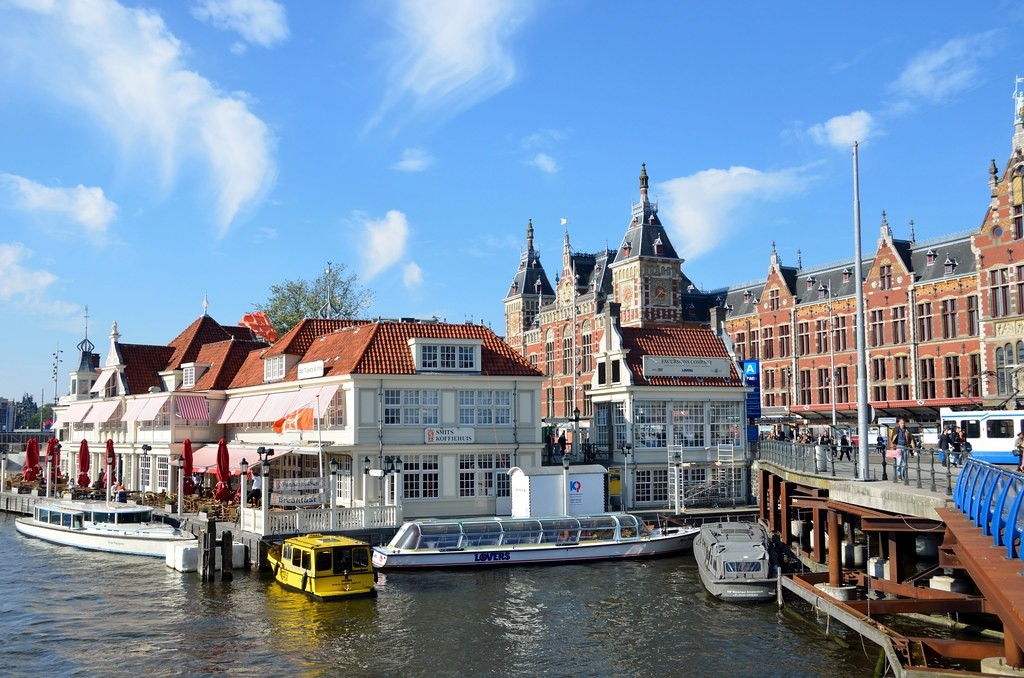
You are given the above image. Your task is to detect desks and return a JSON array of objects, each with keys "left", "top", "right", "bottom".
[{"left": 51, "top": 484, "right": 141, "bottom": 502}]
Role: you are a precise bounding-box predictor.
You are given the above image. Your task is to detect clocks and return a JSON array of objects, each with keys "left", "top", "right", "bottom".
[{"left": 650, "top": 278, "right": 671, "bottom": 305}]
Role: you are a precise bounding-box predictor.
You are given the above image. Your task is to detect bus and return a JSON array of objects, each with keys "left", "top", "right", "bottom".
[{"left": 938, "top": 407, "right": 1024, "bottom": 464}]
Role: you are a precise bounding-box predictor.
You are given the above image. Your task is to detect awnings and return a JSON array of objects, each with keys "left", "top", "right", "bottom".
[
  {"left": 176, "top": 396, "right": 210, "bottom": 420},
  {"left": 121, "top": 397, "right": 170, "bottom": 421},
  {"left": 213, "top": 385, "right": 339, "bottom": 424},
  {"left": 84, "top": 401, "right": 121, "bottom": 423},
  {"left": 57, "top": 403, "right": 92, "bottom": 423},
  {"left": 191, "top": 445, "right": 292, "bottom": 476}
]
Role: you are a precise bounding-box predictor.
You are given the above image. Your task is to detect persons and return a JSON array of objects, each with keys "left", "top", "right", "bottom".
[
  {"left": 35, "top": 466, "right": 122, "bottom": 499},
  {"left": 877, "top": 418, "right": 1024, "bottom": 481},
  {"left": 767, "top": 530, "right": 789, "bottom": 574},
  {"left": 191, "top": 473, "right": 203, "bottom": 498},
  {"left": 759, "top": 426, "right": 856, "bottom": 461},
  {"left": 546, "top": 430, "right": 568, "bottom": 456},
  {"left": 247, "top": 470, "right": 262, "bottom": 505}
]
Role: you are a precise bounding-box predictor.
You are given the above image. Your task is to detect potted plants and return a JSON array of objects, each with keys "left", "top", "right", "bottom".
[
  {"left": 62, "top": 491, "right": 73, "bottom": 500},
  {"left": 199, "top": 506, "right": 213, "bottom": 519},
  {"left": 30, "top": 483, "right": 42, "bottom": 496},
  {"left": 164, "top": 499, "right": 177, "bottom": 513}
]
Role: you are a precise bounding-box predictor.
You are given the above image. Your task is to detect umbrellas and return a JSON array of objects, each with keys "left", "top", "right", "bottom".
[
  {"left": 77, "top": 439, "right": 91, "bottom": 485},
  {"left": 45, "top": 438, "right": 62, "bottom": 481},
  {"left": 183, "top": 438, "right": 194, "bottom": 480},
  {"left": 23, "top": 437, "right": 40, "bottom": 481},
  {"left": 215, "top": 438, "right": 231, "bottom": 502},
  {"left": 102, "top": 439, "right": 117, "bottom": 487}
]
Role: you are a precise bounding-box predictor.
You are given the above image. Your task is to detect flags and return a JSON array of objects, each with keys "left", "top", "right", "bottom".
[{"left": 271, "top": 398, "right": 317, "bottom": 434}]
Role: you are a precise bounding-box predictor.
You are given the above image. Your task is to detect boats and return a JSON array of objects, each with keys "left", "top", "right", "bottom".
[
  {"left": 267, "top": 533, "right": 378, "bottom": 602},
  {"left": 370, "top": 514, "right": 700, "bottom": 568},
  {"left": 693, "top": 522, "right": 778, "bottom": 601},
  {"left": 15, "top": 499, "right": 198, "bottom": 557}
]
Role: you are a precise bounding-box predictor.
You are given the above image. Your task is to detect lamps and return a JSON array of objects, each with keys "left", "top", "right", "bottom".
[
  {"left": 327, "top": 458, "right": 338, "bottom": 475},
  {"left": 262, "top": 460, "right": 270, "bottom": 477},
  {"left": 47, "top": 450, "right": 53, "bottom": 462},
  {"left": 2, "top": 449, "right": 7, "bottom": 460},
  {"left": 106, "top": 452, "right": 114, "bottom": 464},
  {"left": 674, "top": 452, "right": 680, "bottom": 467},
  {"left": 384, "top": 456, "right": 402, "bottom": 474},
  {"left": 240, "top": 458, "right": 249, "bottom": 475},
  {"left": 362, "top": 456, "right": 371, "bottom": 475},
  {"left": 562, "top": 454, "right": 570, "bottom": 471}
]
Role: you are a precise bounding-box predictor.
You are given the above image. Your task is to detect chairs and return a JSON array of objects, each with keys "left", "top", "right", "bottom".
[{"left": 146, "top": 489, "right": 239, "bottom": 522}]
